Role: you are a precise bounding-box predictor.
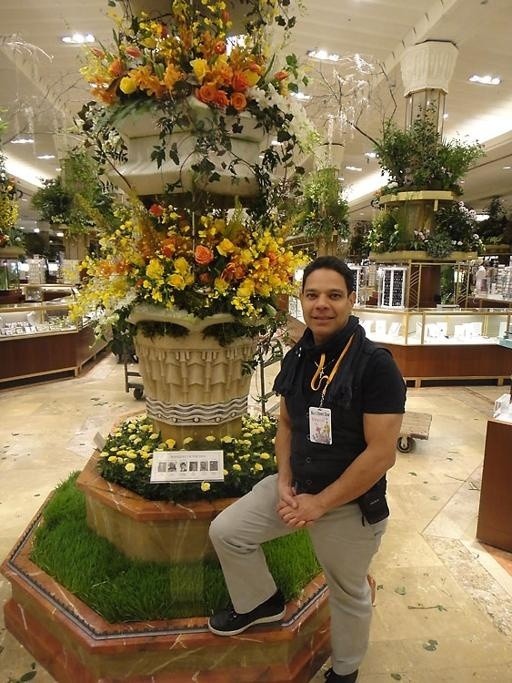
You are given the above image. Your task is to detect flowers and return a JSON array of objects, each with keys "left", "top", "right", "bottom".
[
  {"left": 101, "top": 409, "right": 280, "bottom": 492},
  {"left": 77, "top": 0, "right": 306, "bottom": 118},
  {"left": 0, "top": 169, "right": 29, "bottom": 248},
  {"left": 362, "top": 199, "right": 487, "bottom": 259},
  {"left": 66, "top": 195, "right": 313, "bottom": 346}
]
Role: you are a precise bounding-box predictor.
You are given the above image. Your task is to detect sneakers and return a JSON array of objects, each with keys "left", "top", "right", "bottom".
[
  {"left": 208, "top": 589, "right": 287, "bottom": 636},
  {"left": 324, "top": 668, "right": 358, "bottom": 683}
]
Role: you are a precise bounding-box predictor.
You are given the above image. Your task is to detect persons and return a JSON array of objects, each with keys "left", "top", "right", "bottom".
[
  {"left": 207, "top": 256, "right": 406, "bottom": 682},
  {"left": 200, "top": 461, "right": 206, "bottom": 471},
  {"left": 180, "top": 462, "right": 187, "bottom": 472},
  {"left": 168, "top": 462, "right": 176, "bottom": 472}
]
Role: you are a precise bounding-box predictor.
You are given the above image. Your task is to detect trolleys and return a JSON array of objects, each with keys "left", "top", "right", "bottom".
[
  {"left": 124, "top": 345, "right": 145, "bottom": 403},
  {"left": 259, "top": 341, "right": 433, "bottom": 453}
]
